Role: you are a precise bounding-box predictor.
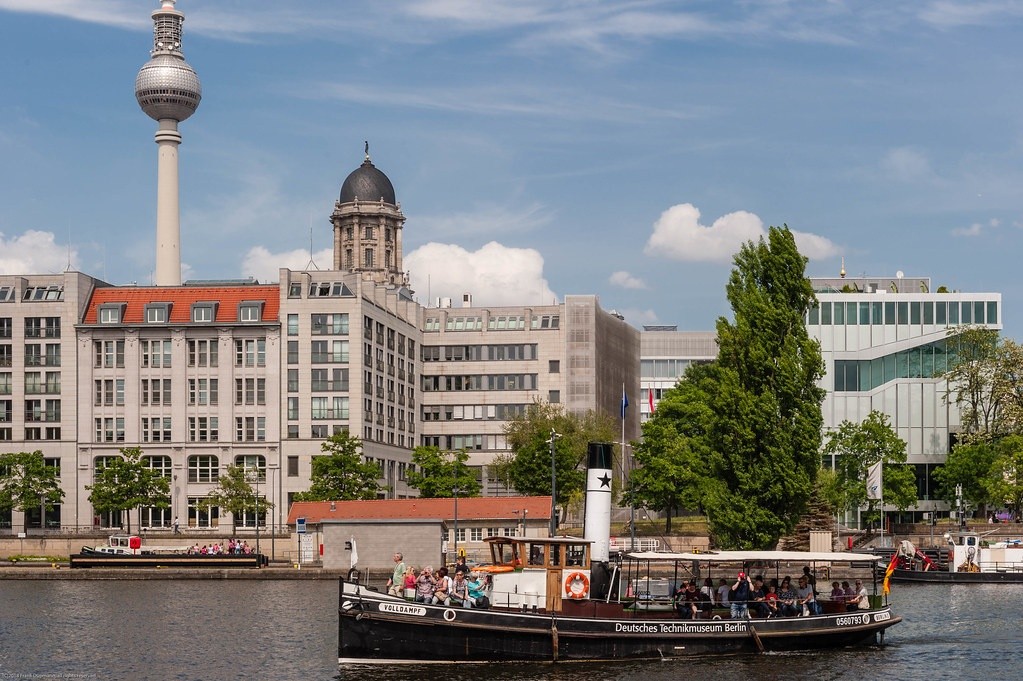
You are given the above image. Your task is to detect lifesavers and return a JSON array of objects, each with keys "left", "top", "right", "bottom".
[
  {"left": 565, "top": 572, "right": 589, "bottom": 598},
  {"left": 459, "top": 549, "right": 465, "bottom": 556},
  {"left": 948, "top": 550, "right": 954, "bottom": 562},
  {"left": 486, "top": 566, "right": 514, "bottom": 572},
  {"left": 471, "top": 566, "right": 491, "bottom": 571}
]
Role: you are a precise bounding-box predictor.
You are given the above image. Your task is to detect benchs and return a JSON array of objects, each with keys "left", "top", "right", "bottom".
[{"left": 623, "top": 609, "right": 759, "bottom": 618}]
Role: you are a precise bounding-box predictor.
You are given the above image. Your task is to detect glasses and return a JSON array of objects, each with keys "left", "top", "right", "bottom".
[
  {"left": 783, "top": 582, "right": 788, "bottom": 584},
  {"left": 799, "top": 581, "right": 803, "bottom": 583},
  {"left": 457, "top": 574, "right": 462, "bottom": 576},
  {"left": 856, "top": 583, "right": 861, "bottom": 585}
]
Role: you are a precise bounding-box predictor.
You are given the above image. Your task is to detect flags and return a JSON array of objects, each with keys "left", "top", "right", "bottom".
[
  {"left": 883, "top": 552, "right": 898, "bottom": 594},
  {"left": 351, "top": 540, "right": 357, "bottom": 565}
]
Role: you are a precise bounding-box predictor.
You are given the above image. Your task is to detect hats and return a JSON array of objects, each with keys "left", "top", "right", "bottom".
[{"left": 737, "top": 572, "right": 745, "bottom": 578}]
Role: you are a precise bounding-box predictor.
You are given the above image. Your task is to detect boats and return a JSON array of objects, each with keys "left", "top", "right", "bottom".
[
  {"left": 338, "top": 508, "right": 901, "bottom": 663},
  {"left": 876, "top": 483, "right": 1023, "bottom": 584},
  {"left": 80, "top": 534, "right": 155, "bottom": 556}
]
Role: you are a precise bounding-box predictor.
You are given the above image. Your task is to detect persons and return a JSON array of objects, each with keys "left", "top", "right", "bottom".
[
  {"left": 989, "top": 512, "right": 998, "bottom": 524},
  {"left": 531, "top": 547, "right": 543, "bottom": 565},
  {"left": 385, "top": 551, "right": 492, "bottom": 609},
  {"left": 186, "top": 537, "right": 255, "bottom": 555},
  {"left": 673, "top": 563, "right": 870, "bottom": 619},
  {"left": 172, "top": 516, "right": 181, "bottom": 535},
  {"left": 625, "top": 581, "right": 632, "bottom": 597}
]
